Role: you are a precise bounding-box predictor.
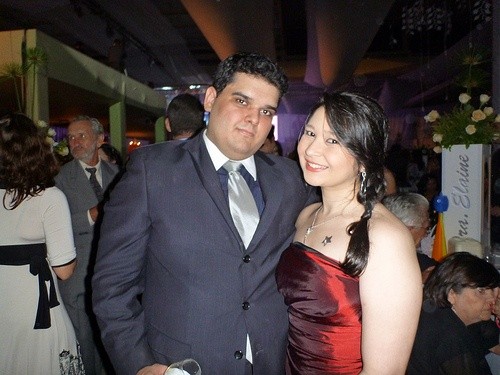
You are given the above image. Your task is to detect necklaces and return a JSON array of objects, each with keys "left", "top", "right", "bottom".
[{"left": 304, "top": 202, "right": 362, "bottom": 238}]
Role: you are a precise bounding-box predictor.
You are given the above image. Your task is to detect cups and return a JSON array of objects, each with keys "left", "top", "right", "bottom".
[
  {"left": 483, "top": 248, "right": 497, "bottom": 265},
  {"left": 162, "top": 358, "right": 201, "bottom": 375}
]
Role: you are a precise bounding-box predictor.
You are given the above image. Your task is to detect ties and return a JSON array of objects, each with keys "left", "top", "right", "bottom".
[
  {"left": 222, "top": 160, "right": 260, "bottom": 251},
  {"left": 86, "top": 168, "right": 102, "bottom": 202}
]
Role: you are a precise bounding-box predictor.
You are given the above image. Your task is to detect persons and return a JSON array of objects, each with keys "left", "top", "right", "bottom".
[
  {"left": 0, "top": 108, "right": 88, "bottom": 375},
  {"left": 407, "top": 251, "right": 500, "bottom": 375},
  {"left": 372, "top": 110, "right": 500, "bottom": 249},
  {"left": 54, "top": 114, "right": 124, "bottom": 375},
  {"left": 276, "top": 91, "right": 424, "bottom": 375},
  {"left": 258, "top": 123, "right": 277, "bottom": 154},
  {"left": 98, "top": 143, "right": 124, "bottom": 173},
  {"left": 129, "top": 93, "right": 205, "bottom": 161},
  {"left": 381, "top": 192, "right": 439, "bottom": 287},
  {"left": 91, "top": 51, "right": 323, "bottom": 375},
  {"left": 272, "top": 141, "right": 283, "bottom": 156}
]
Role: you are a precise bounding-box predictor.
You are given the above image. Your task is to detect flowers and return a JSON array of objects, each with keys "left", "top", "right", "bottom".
[
  {"left": 34, "top": 120, "right": 72, "bottom": 158},
  {"left": 422, "top": 92, "right": 500, "bottom": 151}
]
